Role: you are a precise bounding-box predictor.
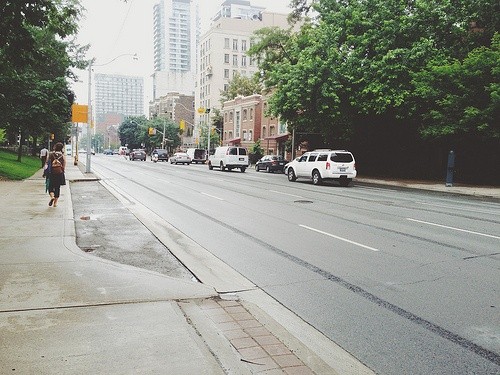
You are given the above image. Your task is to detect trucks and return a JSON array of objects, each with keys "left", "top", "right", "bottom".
[{"left": 187, "top": 147, "right": 206, "bottom": 164}]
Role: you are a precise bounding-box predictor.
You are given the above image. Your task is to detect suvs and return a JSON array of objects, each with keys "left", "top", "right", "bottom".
[
  {"left": 284, "top": 149, "right": 357, "bottom": 186},
  {"left": 207, "top": 146, "right": 249, "bottom": 173}
]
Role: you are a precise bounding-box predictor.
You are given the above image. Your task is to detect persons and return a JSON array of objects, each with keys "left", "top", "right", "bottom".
[
  {"left": 39, "top": 146, "right": 49, "bottom": 168},
  {"left": 42, "top": 142, "right": 66, "bottom": 207}
]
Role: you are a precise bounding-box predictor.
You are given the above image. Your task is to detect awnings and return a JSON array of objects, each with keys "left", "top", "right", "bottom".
[
  {"left": 265, "top": 133, "right": 288, "bottom": 141},
  {"left": 225, "top": 137, "right": 241, "bottom": 143}
]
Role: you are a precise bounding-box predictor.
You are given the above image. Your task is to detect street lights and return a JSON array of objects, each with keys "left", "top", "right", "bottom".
[
  {"left": 131, "top": 121, "right": 165, "bottom": 149},
  {"left": 172, "top": 102, "right": 210, "bottom": 160},
  {"left": 86, "top": 52, "right": 139, "bottom": 174}
]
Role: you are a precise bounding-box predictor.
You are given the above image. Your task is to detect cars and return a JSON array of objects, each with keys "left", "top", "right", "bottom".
[
  {"left": 169, "top": 148, "right": 191, "bottom": 165},
  {"left": 102, "top": 146, "right": 131, "bottom": 156},
  {"left": 150, "top": 149, "right": 169, "bottom": 163},
  {"left": 129, "top": 148, "right": 146, "bottom": 161},
  {"left": 255, "top": 155, "right": 290, "bottom": 173}
]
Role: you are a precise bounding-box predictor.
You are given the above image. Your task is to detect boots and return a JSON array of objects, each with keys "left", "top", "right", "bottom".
[
  {"left": 49, "top": 191, "right": 55, "bottom": 206},
  {"left": 54, "top": 197, "right": 58, "bottom": 207}
]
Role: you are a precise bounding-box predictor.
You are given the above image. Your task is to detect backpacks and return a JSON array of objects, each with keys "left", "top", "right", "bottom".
[{"left": 49, "top": 152, "right": 64, "bottom": 175}]
